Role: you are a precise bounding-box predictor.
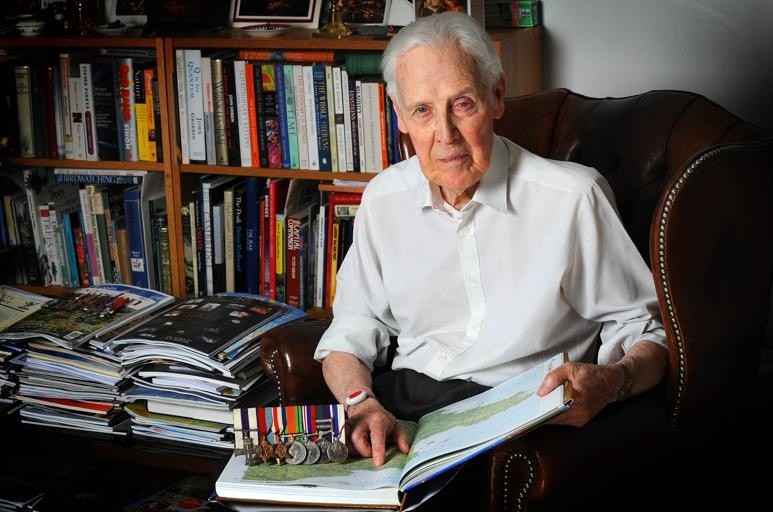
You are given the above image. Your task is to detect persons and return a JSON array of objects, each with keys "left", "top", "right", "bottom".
[{"left": 314, "top": 11, "right": 675, "bottom": 510}]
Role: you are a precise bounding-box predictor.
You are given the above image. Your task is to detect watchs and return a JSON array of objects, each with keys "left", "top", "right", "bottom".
[{"left": 609, "top": 361, "right": 634, "bottom": 403}]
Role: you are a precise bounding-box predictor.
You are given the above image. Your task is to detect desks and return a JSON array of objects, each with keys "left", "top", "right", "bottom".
[{"left": 2, "top": 404, "right": 237, "bottom": 509}]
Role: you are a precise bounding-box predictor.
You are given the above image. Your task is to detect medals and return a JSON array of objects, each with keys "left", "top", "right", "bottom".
[{"left": 233, "top": 403, "right": 350, "bottom": 467}]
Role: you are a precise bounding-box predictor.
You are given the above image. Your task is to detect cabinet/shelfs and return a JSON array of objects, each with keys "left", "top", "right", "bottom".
[{"left": 2, "top": 22, "right": 542, "bottom": 298}]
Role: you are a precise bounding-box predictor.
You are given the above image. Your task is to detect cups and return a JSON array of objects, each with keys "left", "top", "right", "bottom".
[{"left": 359, "top": 23, "right": 387, "bottom": 40}]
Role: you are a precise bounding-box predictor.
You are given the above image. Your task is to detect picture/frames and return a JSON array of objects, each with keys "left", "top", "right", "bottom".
[{"left": 228, "top": 0, "right": 322, "bottom": 29}]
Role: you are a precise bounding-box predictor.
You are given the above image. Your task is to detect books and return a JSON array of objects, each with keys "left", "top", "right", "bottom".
[
  {"left": 209, "top": 352, "right": 574, "bottom": 512},
  {"left": 1, "top": 1, "right": 539, "bottom": 448}
]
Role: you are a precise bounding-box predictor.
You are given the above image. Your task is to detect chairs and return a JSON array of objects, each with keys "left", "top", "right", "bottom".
[{"left": 258, "top": 89, "right": 763, "bottom": 512}]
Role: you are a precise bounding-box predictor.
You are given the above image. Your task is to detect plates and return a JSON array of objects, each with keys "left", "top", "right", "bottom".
[
  {"left": 239, "top": 24, "right": 291, "bottom": 39},
  {"left": 94, "top": 22, "right": 137, "bottom": 35}
]
